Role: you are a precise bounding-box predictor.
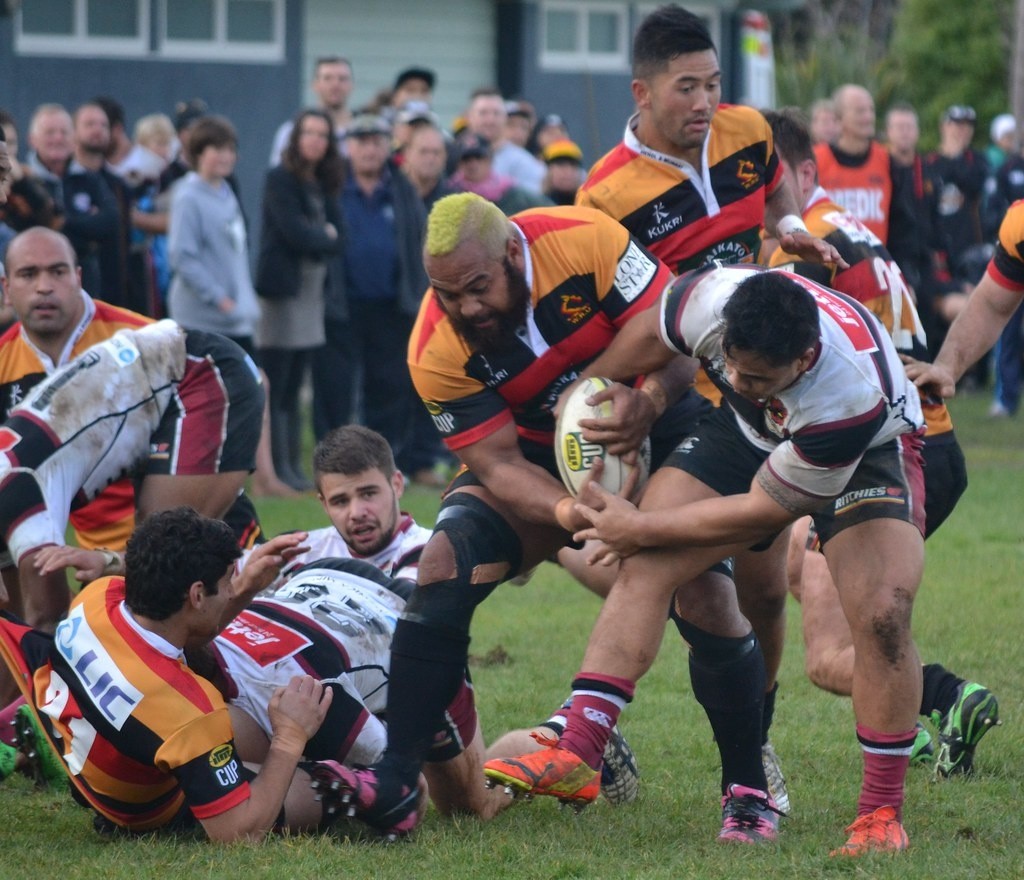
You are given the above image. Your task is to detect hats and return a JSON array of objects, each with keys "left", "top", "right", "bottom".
[
  {"left": 395, "top": 103, "right": 438, "bottom": 128},
  {"left": 540, "top": 140, "right": 582, "bottom": 166},
  {"left": 343, "top": 115, "right": 389, "bottom": 137},
  {"left": 170, "top": 97, "right": 208, "bottom": 131},
  {"left": 942, "top": 103, "right": 978, "bottom": 127}
]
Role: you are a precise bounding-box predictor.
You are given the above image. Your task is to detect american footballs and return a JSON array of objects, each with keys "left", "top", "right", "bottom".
[{"left": 551, "top": 372, "right": 657, "bottom": 520}]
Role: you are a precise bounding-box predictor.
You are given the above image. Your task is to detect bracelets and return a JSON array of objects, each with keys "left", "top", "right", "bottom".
[{"left": 98, "top": 549, "right": 123, "bottom": 576}]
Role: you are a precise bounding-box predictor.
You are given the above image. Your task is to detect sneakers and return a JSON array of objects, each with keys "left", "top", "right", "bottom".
[
  {"left": 8, "top": 705, "right": 70, "bottom": 797},
  {"left": 908, "top": 722, "right": 932, "bottom": 768},
  {"left": 483, "top": 731, "right": 604, "bottom": 816},
  {"left": 932, "top": 680, "right": 1004, "bottom": 783},
  {"left": 828, "top": 805, "right": 907, "bottom": 860},
  {"left": 310, "top": 756, "right": 419, "bottom": 842},
  {"left": 715, "top": 784, "right": 789, "bottom": 847},
  {"left": 547, "top": 695, "right": 639, "bottom": 809},
  {"left": 0, "top": 741, "right": 26, "bottom": 779},
  {"left": 761, "top": 740, "right": 790, "bottom": 816}
]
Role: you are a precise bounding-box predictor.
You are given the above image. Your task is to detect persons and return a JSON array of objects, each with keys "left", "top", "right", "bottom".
[{"left": 0, "top": 3, "right": 1024, "bottom": 858}]
